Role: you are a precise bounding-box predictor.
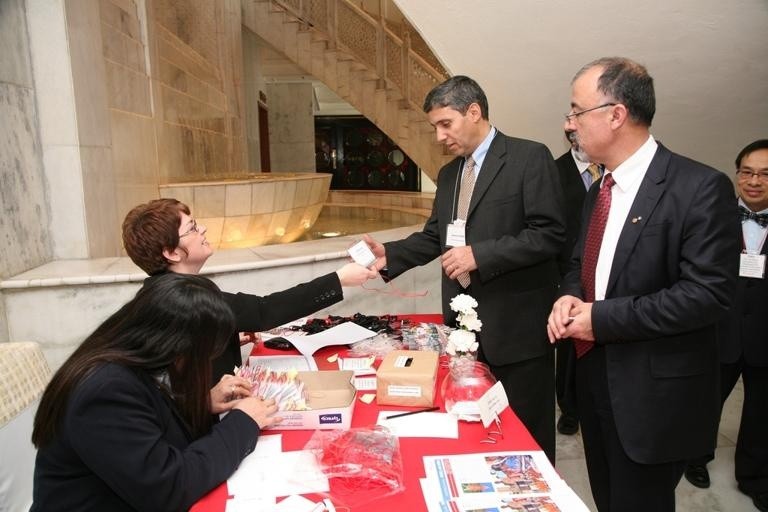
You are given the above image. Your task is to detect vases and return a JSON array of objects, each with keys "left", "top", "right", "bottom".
[{"left": 446, "top": 351, "right": 477, "bottom": 384}]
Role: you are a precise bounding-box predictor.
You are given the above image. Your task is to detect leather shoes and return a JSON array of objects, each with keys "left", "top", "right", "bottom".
[
  {"left": 684, "top": 462, "right": 710, "bottom": 490},
  {"left": 556, "top": 412, "right": 580, "bottom": 436},
  {"left": 737, "top": 482, "right": 765, "bottom": 507}
]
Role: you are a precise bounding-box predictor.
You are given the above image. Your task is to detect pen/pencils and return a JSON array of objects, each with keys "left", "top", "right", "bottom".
[{"left": 382, "top": 407, "right": 440, "bottom": 423}]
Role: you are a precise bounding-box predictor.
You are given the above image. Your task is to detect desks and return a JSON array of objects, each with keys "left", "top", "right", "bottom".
[{"left": 188, "top": 313, "right": 562, "bottom": 512}]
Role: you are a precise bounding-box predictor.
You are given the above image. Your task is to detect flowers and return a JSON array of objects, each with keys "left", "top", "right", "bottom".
[{"left": 444, "top": 293, "right": 482, "bottom": 380}]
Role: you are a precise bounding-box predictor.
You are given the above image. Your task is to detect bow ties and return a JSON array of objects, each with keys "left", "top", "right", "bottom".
[{"left": 741, "top": 207, "right": 765, "bottom": 227}]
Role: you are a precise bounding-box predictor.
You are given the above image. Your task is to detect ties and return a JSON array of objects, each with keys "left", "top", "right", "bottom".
[
  {"left": 573, "top": 174, "right": 616, "bottom": 357},
  {"left": 457, "top": 155, "right": 478, "bottom": 289},
  {"left": 589, "top": 163, "right": 601, "bottom": 183}
]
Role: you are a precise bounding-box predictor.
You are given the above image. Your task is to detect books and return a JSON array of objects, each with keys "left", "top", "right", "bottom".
[{"left": 419, "top": 450, "right": 590, "bottom": 512}]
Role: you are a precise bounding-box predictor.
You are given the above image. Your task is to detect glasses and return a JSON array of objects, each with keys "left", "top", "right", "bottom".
[
  {"left": 734, "top": 170, "right": 763, "bottom": 179},
  {"left": 178, "top": 218, "right": 200, "bottom": 240},
  {"left": 564, "top": 103, "right": 619, "bottom": 123}
]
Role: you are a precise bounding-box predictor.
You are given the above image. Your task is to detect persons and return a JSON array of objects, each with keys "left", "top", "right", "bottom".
[
  {"left": 124, "top": 199, "right": 379, "bottom": 391},
  {"left": 552, "top": 115, "right": 612, "bottom": 436},
  {"left": 544, "top": 55, "right": 743, "bottom": 512},
  {"left": 687, "top": 140, "right": 768, "bottom": 512},
  {"left": 358, "top": 76, "right": 571, "bottom": 468},
  {"left": 27, "top": 275, "right": 283, "bottom": 512}
]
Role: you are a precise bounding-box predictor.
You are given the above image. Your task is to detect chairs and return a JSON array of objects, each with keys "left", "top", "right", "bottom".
[{"left": 0, "top": 342, "right": 55, "bottom": 511}]
